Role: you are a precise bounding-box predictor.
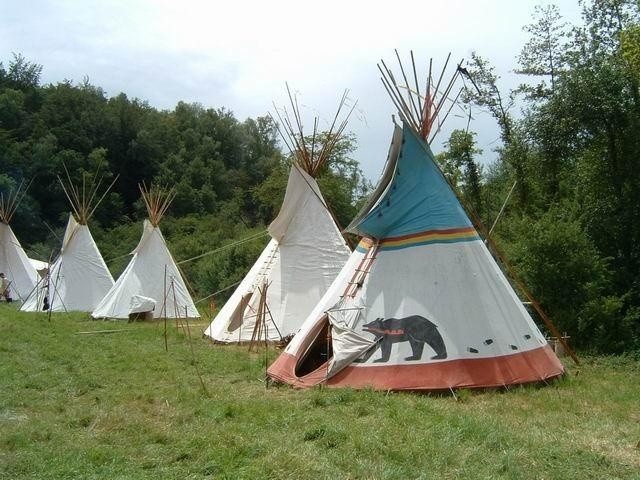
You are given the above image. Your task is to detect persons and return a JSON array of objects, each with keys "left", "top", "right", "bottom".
[{"left": 0, "top": 271, "right": 14, "bottom": 304}]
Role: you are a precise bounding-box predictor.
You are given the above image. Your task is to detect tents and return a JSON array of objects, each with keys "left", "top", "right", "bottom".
[
  {"left": 204, "top": 158, "right": 354, "bottom": 344},
  {"left": 19, "top": 212, "right": 116, "bottom": 313},
  {"left": 91, "top": 217, "right": 203, "bottom": 320},
  {"left": 0, "top": 214, "right": 43, "bottom": 302},
  {"left": 265, "top": 114, "right": 568, "bottom": 391}
]
